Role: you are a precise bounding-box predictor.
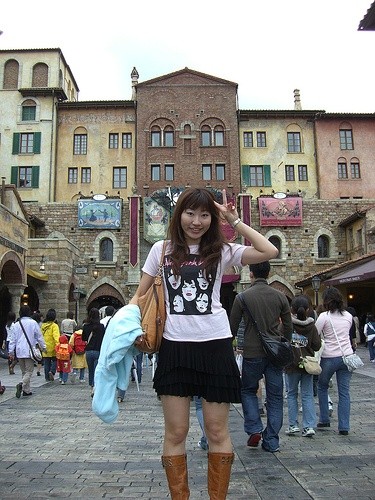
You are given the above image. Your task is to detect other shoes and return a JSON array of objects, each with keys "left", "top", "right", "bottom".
[
  {"left": 80, "top": 380, "right": 87, "bottom": 382},
  {"left": 16, "top": 383, "right": 22, "bottom": 398},
  {"left": 317, "top": 423, "right": 330, "bottom": 429},
  {"left": 0, "top": 381, "right": 5, "bottom": 394},
  {"left": 258, "top": 408, "right": 265, "bottom": 416},
  {"left": 61, "top": 381, "right": 66, "bottom": 384},
  {"left": 9, "top": 363, "right": 16, "bottom": 374},
  {"left": 37, "top": 372, "right": 40, "bottom": 376},
  {"left": 49, "top": 372, "right": 54, "bottom": 380},
  {"left": 247, "top": 433, "right": 261, "bottom": 447},
  {"left": 23, "top": 391, "right": 32, "bottom": 396},
  {"left": 46, "top": 378, "right": 50, "bottom": 381},
  {"left": 339, "top": 431, "right": 349, "bottom": 435},
  {"left": 71, "top": 373, "right": 76, "bottom": 382},
  {"left": 59, "top": 378, "right": 62, "bottom": 381}
]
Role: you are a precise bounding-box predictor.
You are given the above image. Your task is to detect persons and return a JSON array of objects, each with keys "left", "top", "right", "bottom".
[
  {"left": 0, "top": 380, "right": 6, "bottom": 395},
  {"left": 127, "top": 187, "right": 279, "bottom": 500},
  {"left": 104, "top": 309, "right": 126, "bottom": 402},
  {"left": 193, "top": 396, "right": 209, "bottom": 450},
  {"left": 229, "top": 261, "right": 293, "bottom": 452},
  {"left": 277, "top": 295, "right": 322, "bottom": 438},
  {"left": 2, "top": 306, "right": 156, "bottom": 385},
  {"left": 8, "top": 305, "right": 47, "bottom": 399},
  {"left": 81, "top": 308, "right": 105, "bottom": 398},
  {"left": 314, "top": 286, "right": 354, "bottom": 435},
  {"left": 234, "top": 284, "right": 375, "bottom": 417}
]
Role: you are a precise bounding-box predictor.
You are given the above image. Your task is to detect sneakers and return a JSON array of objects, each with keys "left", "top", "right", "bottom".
[
  {"left": 302, "top": 428, "right": 315, "bottom": 436},
  {"left": 285, "top": 426, "right": 300, "bottom": 435},
  {"left": 328, "top": 404, "right": 333, "bottom": 417},
  {"left": 198, "top": 441, "right": 202, "bottom": 448}
]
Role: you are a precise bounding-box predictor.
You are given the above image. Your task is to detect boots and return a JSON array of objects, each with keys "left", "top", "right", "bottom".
[
  {"left": 161, "top": 453, "right": 190, "bottom": 500},
  {"left": 206, "top": 452, "right": 234, "bottom": 500}
]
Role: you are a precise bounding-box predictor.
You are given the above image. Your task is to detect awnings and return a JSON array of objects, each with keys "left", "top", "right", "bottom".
[{"left": 323, "top": 258, "right": 375, "bottom": 286}]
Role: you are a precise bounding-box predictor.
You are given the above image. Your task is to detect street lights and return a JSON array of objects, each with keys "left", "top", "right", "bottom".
[
  {"left": 311, "top": 275, "right": 321, "bottom": 306},
  {"left": 72, "top": 288, "right": 81, "bottom": 323}
]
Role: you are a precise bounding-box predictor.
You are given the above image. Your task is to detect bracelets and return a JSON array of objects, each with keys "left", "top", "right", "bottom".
[{"left": 231, "top": 217, "right": 241, "bottom": 228}]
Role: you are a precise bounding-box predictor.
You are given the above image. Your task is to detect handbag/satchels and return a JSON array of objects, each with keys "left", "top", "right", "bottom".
[
  {"left": 262, "top": 334, "right": 295, "bottom": 366},
  {"left": 1, "top": 340, "right": 6, "bottom": 349},
  {"left": 30, "top": 346, "right": 42, "bottom": 363},
  {"left": 301, "top": 356, "right": 322, "bottom": 375},
  {"left": 343, "top": 354, "right": 364, "bottom": 372},
  {"left": 135, "top": 239, "right": 167, "bottom": 353}
]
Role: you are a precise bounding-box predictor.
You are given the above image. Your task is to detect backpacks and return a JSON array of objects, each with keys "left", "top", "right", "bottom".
[
  {"left": 73, "top": 332, "right": 88, "bottom": 354},
  {"left": 56, "top": 344, "right": 70, "bottom": 360}
]
